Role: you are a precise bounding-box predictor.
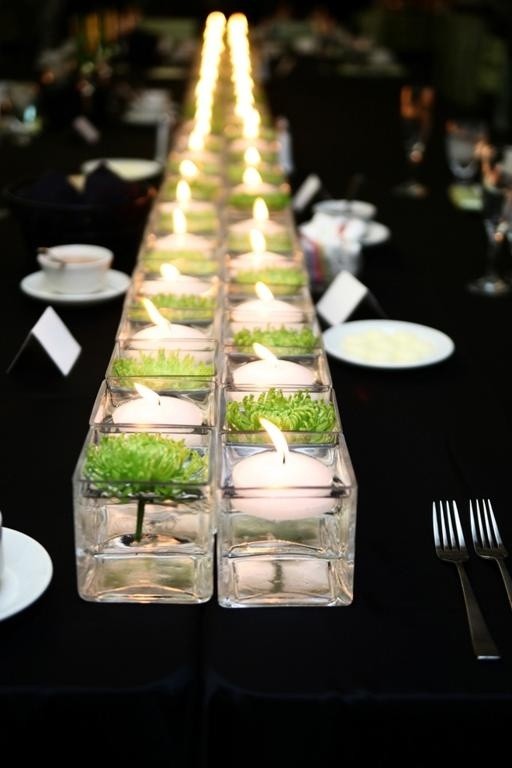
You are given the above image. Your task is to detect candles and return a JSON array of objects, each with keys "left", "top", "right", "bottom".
[
  {"left": 230, "top": 353, "right": 317, "bottom": 393},
  {"left": 228, "top": 443, "right": 341, "bottom": 546},
  {"left": 132, "top": 321, "right": 203, "bottom": 355},
  {"left": 113, "top": 389, "right": 206, "bottom": 430},
  {"left": 129, "top": 1, "right": 322, "bottom": 325}
]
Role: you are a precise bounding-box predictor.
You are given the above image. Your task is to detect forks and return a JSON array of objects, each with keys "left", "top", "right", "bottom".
[{"left": 428, "top": 494, "right": 510, "bottom": 665}]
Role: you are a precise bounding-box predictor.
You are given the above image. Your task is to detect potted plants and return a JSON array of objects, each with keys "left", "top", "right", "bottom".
[
  {"left": 109, "top": 353, "right": 217, "bottom": 385},
  {"left": 75, "top": 430, "right": 217, "bottom": 610},
  {"left": 220, "top": 390, "right": 334, "bottom": 432},
  {"left": 230, "top": 326, "right": 318, "bottom": 355}
]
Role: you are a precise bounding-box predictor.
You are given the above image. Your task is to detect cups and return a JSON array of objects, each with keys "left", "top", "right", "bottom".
[
  {"left": 73, "top": 173, "right": 363, "bottom": 610},
  {"left": 309, "top": 198, "right": 374, "bottom": 238},
  {"left": 37, "top": 244, "right": 112, "bottom": 294}
]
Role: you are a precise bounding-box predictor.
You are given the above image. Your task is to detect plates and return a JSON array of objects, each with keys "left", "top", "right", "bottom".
[
  {"left": 294, "top": 220, "right": 390, "bottom": 250},
  {"left": 318, "top": 320, "right": 454, "bottom": 374},
  {"left": 1, "top": 525, "right": 55, "bottom": 630},
  {"left": 21, "top": 273, "right": 131, "bottom": 307}
]
